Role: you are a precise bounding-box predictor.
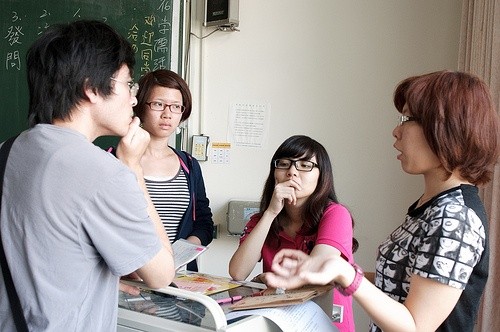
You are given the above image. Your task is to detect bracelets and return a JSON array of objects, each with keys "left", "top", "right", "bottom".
[{"left": 336, "top": 263, "right": 364, "bottom": 297}]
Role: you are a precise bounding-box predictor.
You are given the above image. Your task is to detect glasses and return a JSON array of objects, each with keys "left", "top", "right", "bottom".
[
  {"left": 397, "top": 115, "right": 416, "bottom": 125},
  {"left": 274, "top": 158, "right": 320, "bottom": 172},
  {"left": 145, "top": 102, "right": 186, "bottom": 114},
  {"left": 111, "top": 77, "right": 139, "bottom": 97}
]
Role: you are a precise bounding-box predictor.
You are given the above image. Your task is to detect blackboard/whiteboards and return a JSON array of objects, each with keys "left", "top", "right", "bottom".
[{"left": 0, "top": 1, "right": 186, "bottom": 151}]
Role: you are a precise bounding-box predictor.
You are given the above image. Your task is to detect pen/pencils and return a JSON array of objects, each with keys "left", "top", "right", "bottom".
[{"left": 216, "top": 295, "right": 242, "bottom": 303}]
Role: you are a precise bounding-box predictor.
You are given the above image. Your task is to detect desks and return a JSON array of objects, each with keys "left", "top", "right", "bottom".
[{"left": 118, "top": 275, "right": 334, "bottom": 332}]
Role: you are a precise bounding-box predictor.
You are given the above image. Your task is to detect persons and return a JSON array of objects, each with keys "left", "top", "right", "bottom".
[
  {"left": 228, "top": 134, "right": 355, "bottom": 332},
  {"left": 261, "top": 70, "right": 500, "bottom": 332},
  {"left": 2, "top": 20, "right": 173, "bottom": 332},
  {"left": 107, "top": 68, "right": 216, "bottom": 272}
]
